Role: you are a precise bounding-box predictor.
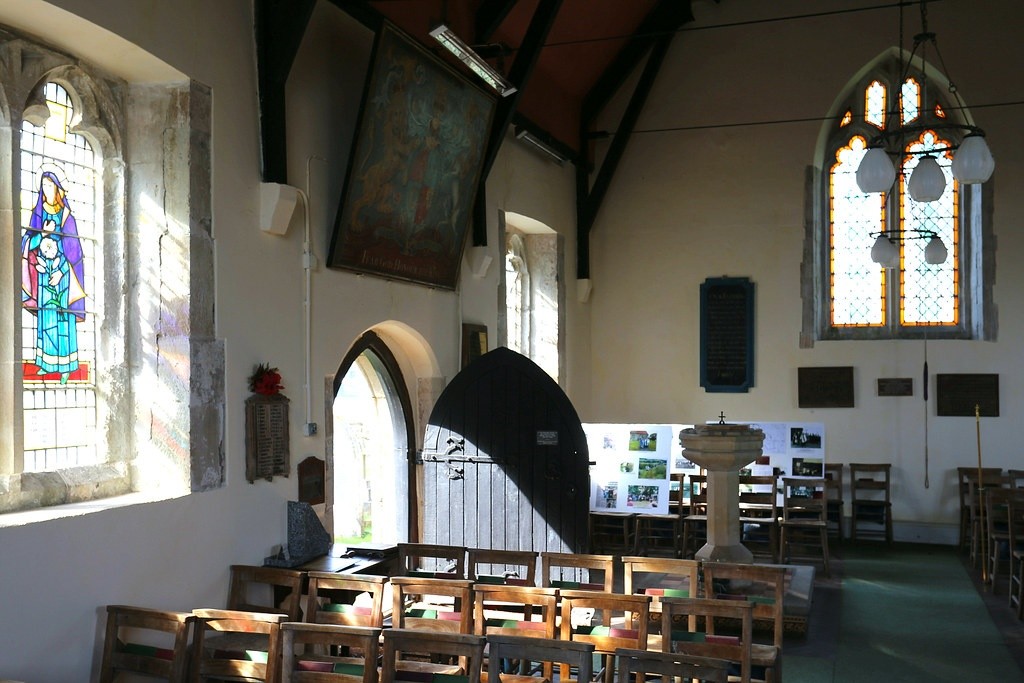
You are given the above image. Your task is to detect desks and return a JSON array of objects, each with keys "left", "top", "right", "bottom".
[{"left": 264, "top": 543, "right": 398, "bottom": 610}]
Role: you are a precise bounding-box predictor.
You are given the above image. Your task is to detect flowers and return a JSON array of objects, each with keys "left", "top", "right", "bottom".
[{"left": 249, "top": 362, "right": 285, "bottom": 396}]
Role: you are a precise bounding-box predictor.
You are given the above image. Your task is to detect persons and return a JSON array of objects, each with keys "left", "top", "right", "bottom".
[
  {"left": 627, "top": 497, "right": 634, "bottom": 506},
  {"left": 625, "top": 463, "right": 631, "bottom": 472},
  {"left": 638, "top": 435, "right": 651, "bottom": 449}
]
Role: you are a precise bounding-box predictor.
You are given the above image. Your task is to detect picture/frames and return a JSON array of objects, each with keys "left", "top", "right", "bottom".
[{"left": 326, "top": 18, "right": 502, "bottom": 292}]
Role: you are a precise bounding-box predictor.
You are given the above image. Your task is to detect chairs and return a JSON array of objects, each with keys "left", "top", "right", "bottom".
[
  {"left": 957, "top": 467, "right": 1024, "bottom": 620},
  {"left": 100, "top": 543, "right": 787, "bottom": 683},
  {"left": 592, "top": 458, "right": 893, "bottom": 579}
]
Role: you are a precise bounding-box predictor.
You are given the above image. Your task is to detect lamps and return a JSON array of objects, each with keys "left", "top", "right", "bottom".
[{"left": 856, "top": 0, "right": 995, "bottom": 267}]
